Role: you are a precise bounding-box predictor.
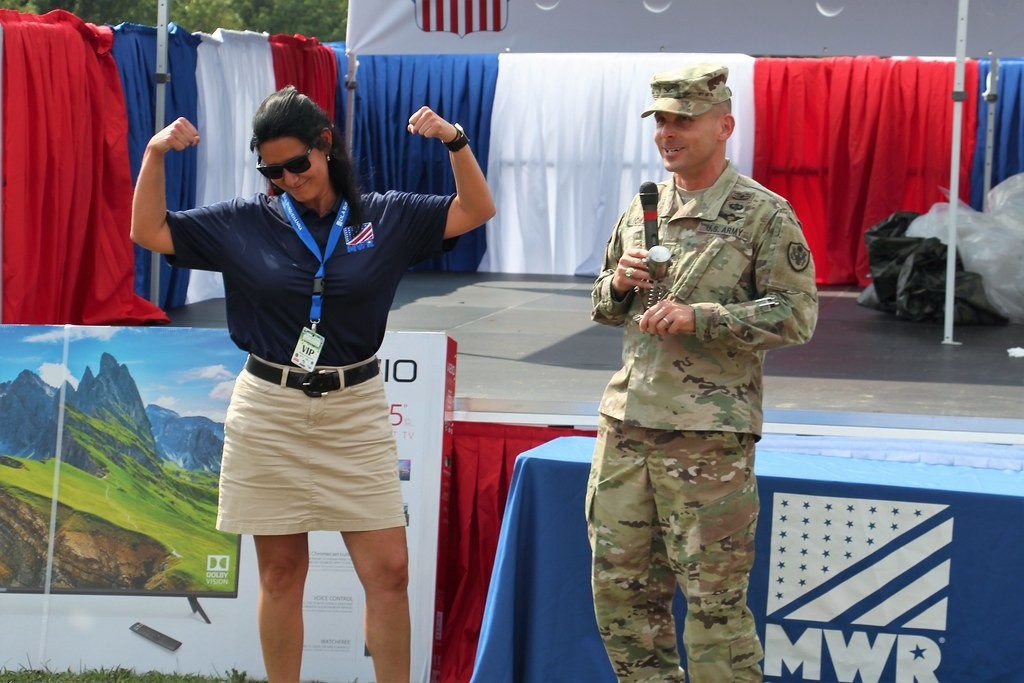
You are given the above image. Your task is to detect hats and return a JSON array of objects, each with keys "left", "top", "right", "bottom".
[{"left": 641, "top": 63, "right": 734, "bottom": 117}]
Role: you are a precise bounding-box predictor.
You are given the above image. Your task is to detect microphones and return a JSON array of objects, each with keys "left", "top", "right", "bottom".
[{"left": 640, "top": 182, "right": 671, "bottom": 282}]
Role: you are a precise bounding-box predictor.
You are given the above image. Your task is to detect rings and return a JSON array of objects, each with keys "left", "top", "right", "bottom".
[
  {"left": 663, "top": 318, "right": 669, "bottom": 325},
  {"left": 625, "top": 268, "right": 633, "bottom": 278}
]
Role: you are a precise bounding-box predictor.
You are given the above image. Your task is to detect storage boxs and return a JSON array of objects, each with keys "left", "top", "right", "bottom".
[{"left": 0, "top": 322, "right": 458, "bottom": 683}]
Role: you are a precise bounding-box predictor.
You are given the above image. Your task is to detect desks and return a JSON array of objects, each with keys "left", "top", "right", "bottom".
[{"left": 470, "top": 434, "right": 1024, "bottom": 683}]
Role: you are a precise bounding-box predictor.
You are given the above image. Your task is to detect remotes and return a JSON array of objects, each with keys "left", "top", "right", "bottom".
[{"left": 130, "top": 622, "right": 182, "bottom": 651}]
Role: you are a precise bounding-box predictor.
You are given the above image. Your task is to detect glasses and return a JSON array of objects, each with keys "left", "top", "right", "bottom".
[{"left": 255, "top": 146, "right": 313, "bottom": 179}]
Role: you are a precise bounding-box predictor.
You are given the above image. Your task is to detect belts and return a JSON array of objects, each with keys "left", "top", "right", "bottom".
[{"left": 246, "top": 355, "right": 379, "bottom": 398}]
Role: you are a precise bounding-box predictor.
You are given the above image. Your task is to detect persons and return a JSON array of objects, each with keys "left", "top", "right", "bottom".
[
  {"left": 586, "top": 64, "right": 818, "bottom": 683},
  {"left": 128, "top": 84, "right": 495, "bottom": 683}
]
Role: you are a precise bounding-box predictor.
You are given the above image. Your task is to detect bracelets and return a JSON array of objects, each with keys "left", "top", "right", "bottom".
[{"left": 444, "top": 131, "right": 458, "bottom": 143}]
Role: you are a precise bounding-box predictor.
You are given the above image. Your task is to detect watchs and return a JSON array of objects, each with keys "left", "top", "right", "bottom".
[{"left": 441, "top": 122, "right": 469, "bottom": 152}]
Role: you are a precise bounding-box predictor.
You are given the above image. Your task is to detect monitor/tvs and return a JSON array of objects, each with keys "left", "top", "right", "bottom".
[{"left": 0, "top": 325, "right": 240, "bottom": 623}]
